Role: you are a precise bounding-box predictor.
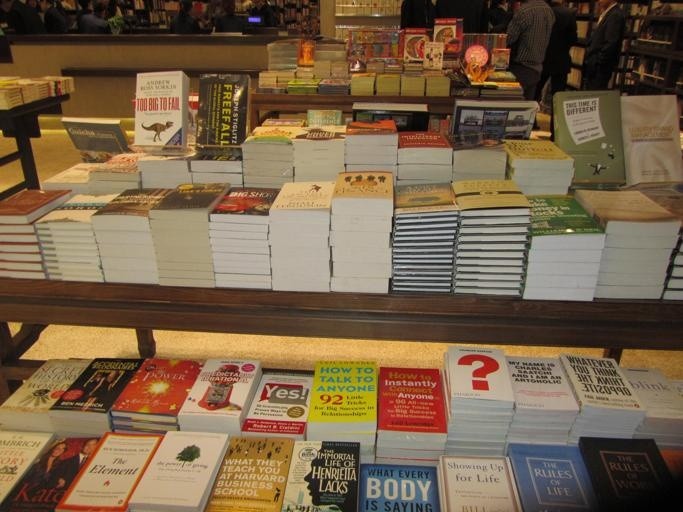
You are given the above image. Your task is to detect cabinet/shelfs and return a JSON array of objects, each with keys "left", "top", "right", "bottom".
[
  {"left": 0, "top": 0, "right": 322, "bottom": 40},
  {"left": 567, "top": 0, "right": 642, "bottom": 90},
  {"left": 617, "top": 0, "right": 682, "bottom": 93}
]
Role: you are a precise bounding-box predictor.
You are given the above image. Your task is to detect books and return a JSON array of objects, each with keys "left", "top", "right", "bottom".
[
  {"left": 2, "top": 76, "right": 74, "bottom": 110},
  {"left": 3, "top": 356, "right": 448, "bottom": 511},
  {"left": 258, "top": 39, "right": 525, "bottom": 97},
  {"left": 2, "top": 91, "right": 683, "bottom": 301},
  {"left": 438, "top": 346, "right": 681, "bottom": 510},
  {"left": 197, "top": 74, "right": 252, "bottom": 146},
  {"left": 134, "top": 71, "right": 190, "bottom": 147},
  {"left": 62, "top": 117, "right": 129, "bottom": 164}
]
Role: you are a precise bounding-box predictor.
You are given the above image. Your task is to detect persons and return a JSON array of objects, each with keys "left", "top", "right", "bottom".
[
  {"left": 401, "top": 0, "right": 625, "bottom": 142},
  {"left": 0, "top": 1, "right": 317, "bottom": 33}
]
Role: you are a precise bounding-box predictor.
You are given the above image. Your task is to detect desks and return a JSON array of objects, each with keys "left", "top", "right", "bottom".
[
  {"left": 249, "top": 88, "right": 524, "bottom": 135},
  {"left": 0, "top": 91, "right": 72, "bottom": 200},
  {"left": 1, "top": 276, "right": 682, "bottom": 399}
]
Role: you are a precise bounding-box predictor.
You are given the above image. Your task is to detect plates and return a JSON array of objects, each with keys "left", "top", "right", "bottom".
[{"left": 465, "top": 44, "right": 488, "bottom": 67}]
